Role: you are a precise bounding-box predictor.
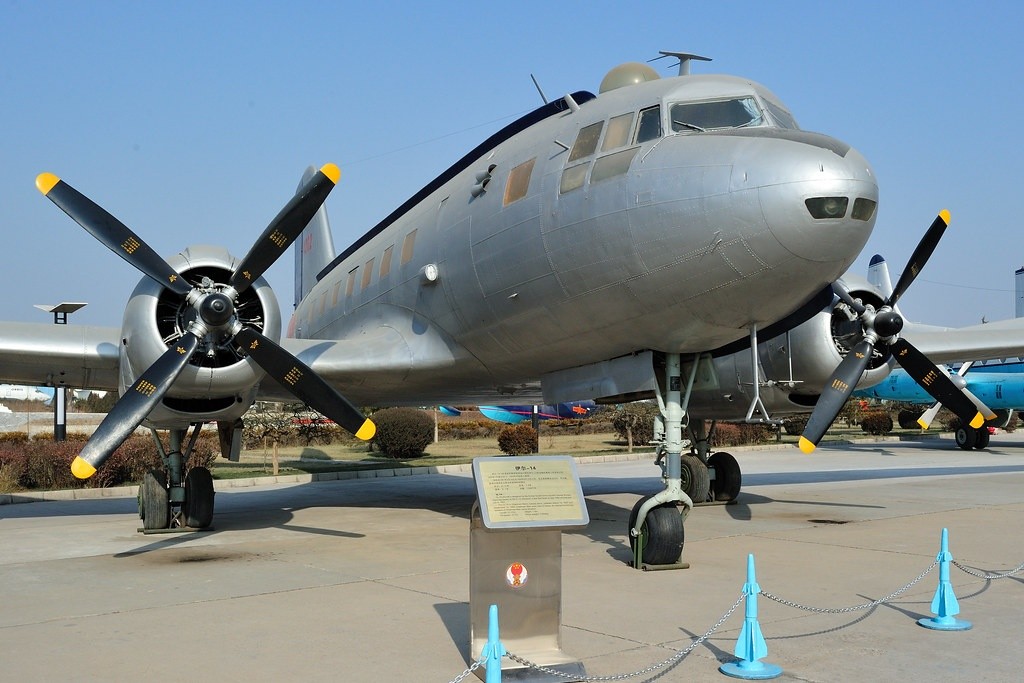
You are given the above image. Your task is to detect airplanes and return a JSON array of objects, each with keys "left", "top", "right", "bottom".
[
  {"left": 847, "top": 359, "right": 1024, "bottom": 451},
  {"left": 1, "top": 48, "right": 1000, "bottom": 573}
]
